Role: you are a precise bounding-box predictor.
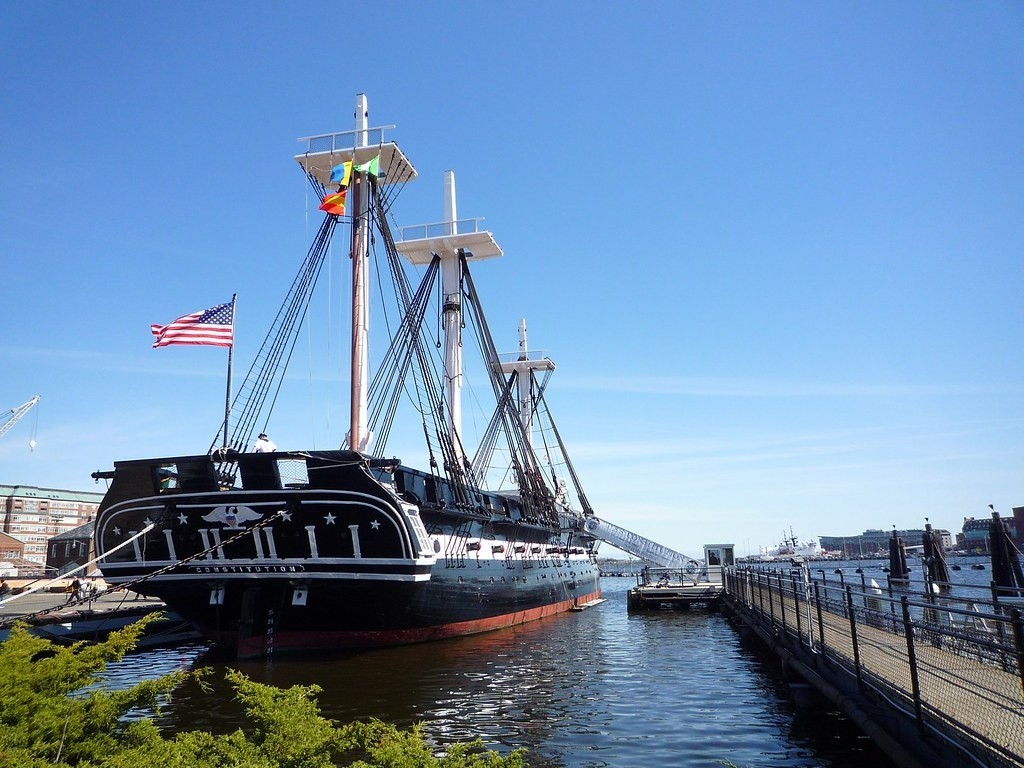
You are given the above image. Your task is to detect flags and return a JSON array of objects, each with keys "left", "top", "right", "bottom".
[
  {"left": 353, "top": 150, "right": 381, "bottom": 176},
  {"left": 318, "top": 189, "right": 346, "bottom": 216},
  {"left": 151, "top": 300, "right": 234, "bottom": 349},
  {"left": 329, "top": 158, "right": 353, "bottom": 186}
]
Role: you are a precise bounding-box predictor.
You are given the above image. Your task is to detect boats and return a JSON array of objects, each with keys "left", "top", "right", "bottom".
[{"left": 89, "top": 98, "right": 608, "bottom": 654}]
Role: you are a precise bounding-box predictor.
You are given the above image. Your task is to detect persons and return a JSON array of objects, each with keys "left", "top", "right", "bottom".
[
  {"left": 65, "top": 575, "right": 99, "bottom": 605},
  {"left": 709, "top": 552, "right": 718, "bottom": 565},
  {"left": 251, "top": 432, "right": 276, "bottom": 453}
]
